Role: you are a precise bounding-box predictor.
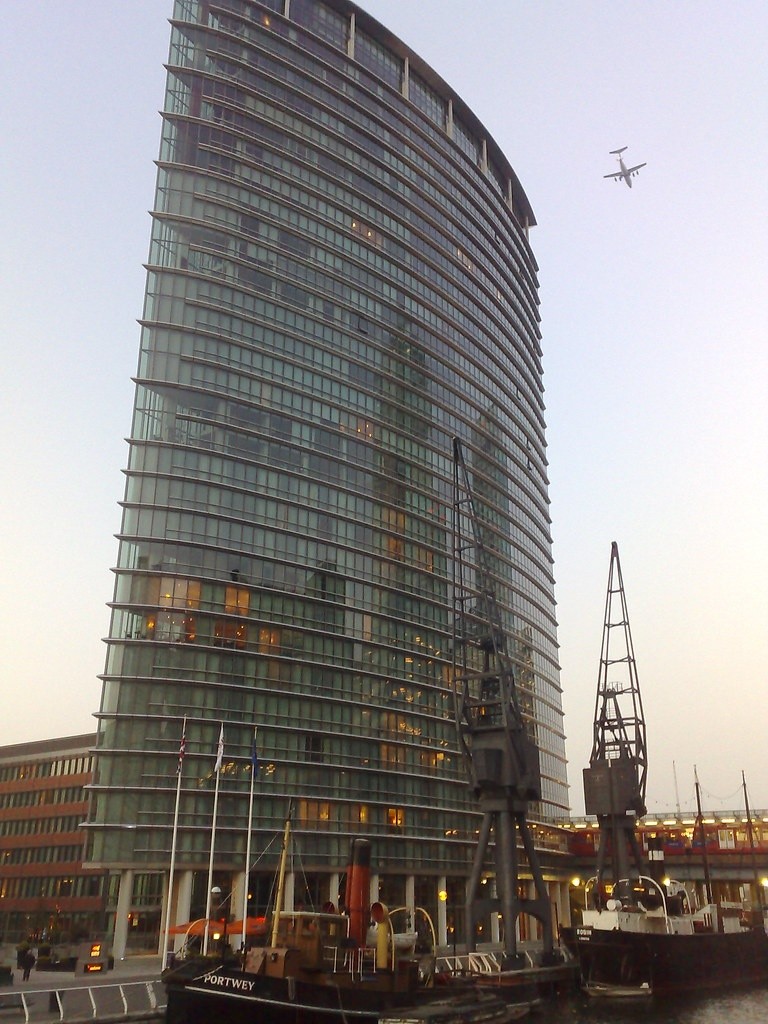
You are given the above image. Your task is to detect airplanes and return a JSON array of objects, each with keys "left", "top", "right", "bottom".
[{"left": 603, "top": 146, "right": 647, "bottom": 189}]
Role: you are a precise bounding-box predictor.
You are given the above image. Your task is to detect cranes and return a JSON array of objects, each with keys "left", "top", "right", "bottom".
[
  {"left": 448, "top": 432, "right": 564, "bottom": 977},
  {"left": 578, "top": 540, "right": 653, "bottom": 918}
]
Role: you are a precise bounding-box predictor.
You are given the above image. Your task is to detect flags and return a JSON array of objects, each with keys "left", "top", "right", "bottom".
[{"left": 175, "top": 725, "right": 261, "bottom": 779}]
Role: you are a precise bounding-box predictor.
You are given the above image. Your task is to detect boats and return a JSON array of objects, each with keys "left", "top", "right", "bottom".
[
  {"left": 159, "top": 821, "right": 545, "bottom": 1024},
  {"left": 557, "top": 767, "right": 767, "bottom": 991},
  {"left": 581, "top": 975, "right": 655, "bottom": 1000}
]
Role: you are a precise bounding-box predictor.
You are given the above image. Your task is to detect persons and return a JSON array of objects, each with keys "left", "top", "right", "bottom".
[{"left": 21, "top": 950, "right": 35, "bottom": 982}]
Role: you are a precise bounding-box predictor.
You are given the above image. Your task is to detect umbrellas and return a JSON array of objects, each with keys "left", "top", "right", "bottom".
[{"left": 165, "top": 918, "right": 269, "bottom": 955}]
